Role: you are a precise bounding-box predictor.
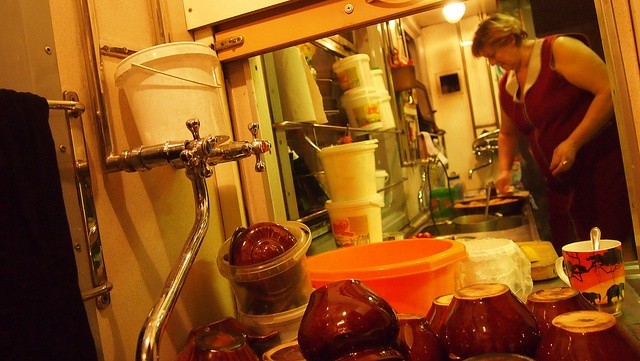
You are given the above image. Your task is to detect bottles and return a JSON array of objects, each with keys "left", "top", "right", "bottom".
[{"left": 429, "top": 164, "right": 452, "bottom": 220}]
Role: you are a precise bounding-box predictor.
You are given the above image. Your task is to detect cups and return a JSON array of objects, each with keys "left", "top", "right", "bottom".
[{"left": 555, "top": 239, "right": 625, "bottom": 319}]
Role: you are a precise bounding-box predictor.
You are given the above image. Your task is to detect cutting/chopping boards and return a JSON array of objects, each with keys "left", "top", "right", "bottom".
[{"left": 515, "top": 240, "right": 560, "bottom": 282}]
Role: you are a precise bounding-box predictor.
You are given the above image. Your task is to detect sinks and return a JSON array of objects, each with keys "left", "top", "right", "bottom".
[{"left": 415, "top": 214, "right": 524, "bottom": 236}]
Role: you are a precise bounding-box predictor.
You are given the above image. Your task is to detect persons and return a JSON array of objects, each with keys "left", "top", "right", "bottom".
[{"left": 471, "top": 13, "right": 638, "bottom": 261}]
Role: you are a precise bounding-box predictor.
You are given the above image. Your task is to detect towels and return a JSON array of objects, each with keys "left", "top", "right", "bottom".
[{"left": 0, "top": 88, "right": 102, "bottom": 361}]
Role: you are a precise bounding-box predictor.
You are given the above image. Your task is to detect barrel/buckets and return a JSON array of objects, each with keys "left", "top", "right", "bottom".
[
  {"left": 325, "top": 200, "right": 384, "bottom": 249},
  {"left": 340, "top": 86, "right": 385, "bottom": 137},
  {"left": 377, "top": 95, "right": 395, "bottom": 131},
  {"left": 237, "top": 302, "right": 309, "bottom": 343},
  {"left": 333, "top": 53, "right": 373, "bottom": 92},
  {"left": 377, "top": 169, "right": 389, "bottom": 208},
  {"left": 372, "top": 67, "right": 391, "bottom": 96},
  {"left": 217, "top": 221, "right": 312, "bottom": 313},
  {"left": 318, "top": 139, "right": 380, "bottom": 203},
  {"left": 114, "top": 42, "right": 231, "bottom": 148}
]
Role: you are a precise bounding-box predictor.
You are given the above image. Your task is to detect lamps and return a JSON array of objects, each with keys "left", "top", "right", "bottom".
[{"left": 440, "top": 2, "right": 465, "bottom": 24}]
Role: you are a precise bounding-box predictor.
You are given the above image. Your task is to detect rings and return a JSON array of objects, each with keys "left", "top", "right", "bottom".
[{"left": 561, "top": 159, "right": 567, "bottom": 165}]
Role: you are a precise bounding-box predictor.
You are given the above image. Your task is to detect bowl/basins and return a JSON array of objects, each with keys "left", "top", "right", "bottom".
[
  {"left": 449, "top": 196, "right": 526, "bottom": 213},
  {"left": 452, "top": 214, "right": 497, "bottom": 232}
]
